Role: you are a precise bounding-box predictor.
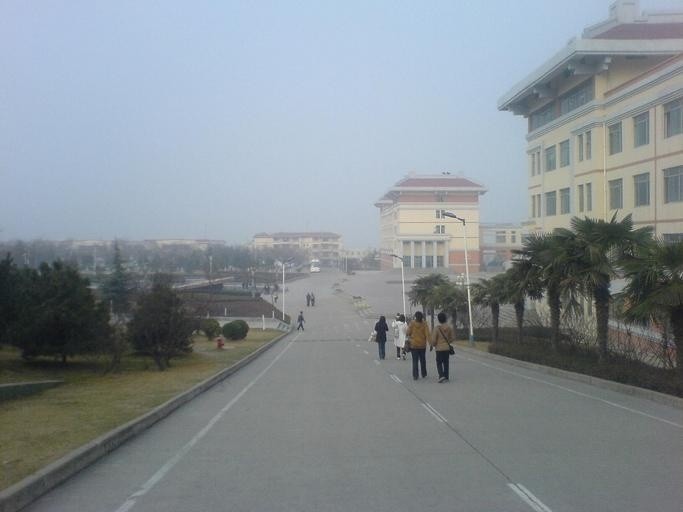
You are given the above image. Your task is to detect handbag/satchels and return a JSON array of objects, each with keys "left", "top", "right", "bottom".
[
  {"left": 394, "top": 322, "right": 399, "bottom": 337},
  {"left": 369, "top": 332, "right": 376, "bottom": 343},
  {"left": 449, "top": 345, "right": 455, "bottom": 355}
]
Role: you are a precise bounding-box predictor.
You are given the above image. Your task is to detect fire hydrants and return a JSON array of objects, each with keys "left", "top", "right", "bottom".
[{"left": 217, "top": 338, "right": 225, "bottom": 349}]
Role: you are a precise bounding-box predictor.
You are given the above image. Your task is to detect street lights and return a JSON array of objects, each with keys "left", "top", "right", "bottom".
[
  {"left": 389, "top": 254, "right": 407, "bottom": 324},
  {"left": 441, "top": 212, "right": 475, "bottom": 344},
  {"left": 282, "top": 257, "right": 295, "bottom": 321}
]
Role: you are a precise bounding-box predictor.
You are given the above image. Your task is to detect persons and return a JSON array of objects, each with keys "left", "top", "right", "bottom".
[
  {"left": 265, "top": 283, "right": 270, "bottom": 294},
  {"left": 306, "top": 292, "right": 310, "bottom": 306},
  {"left": 406, "top": 311, "right": 432, "bottom": 380},
  {"left": 297, "top": 310, "right": 306, "bottom": 331},
  {"left": 431, "top": 313, "right": 454, "bottom": 383},
  {"left": 274, "top": 293, "right": 278, "bottom": 302},
  {"left": 374, "top": 312, "right": 410, "bottom": 360},
  {"left": 311, "top": 292, "right": 315, "bottom": 306}
]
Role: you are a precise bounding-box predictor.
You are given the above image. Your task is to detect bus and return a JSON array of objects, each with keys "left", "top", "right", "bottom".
[{"left": 310, "top": 259, "right": 321, "bottom": 273}]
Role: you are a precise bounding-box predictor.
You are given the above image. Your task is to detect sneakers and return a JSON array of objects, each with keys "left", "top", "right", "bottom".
[{"left": 439, "top": 377, "right": 449, "bottom": 383}]
